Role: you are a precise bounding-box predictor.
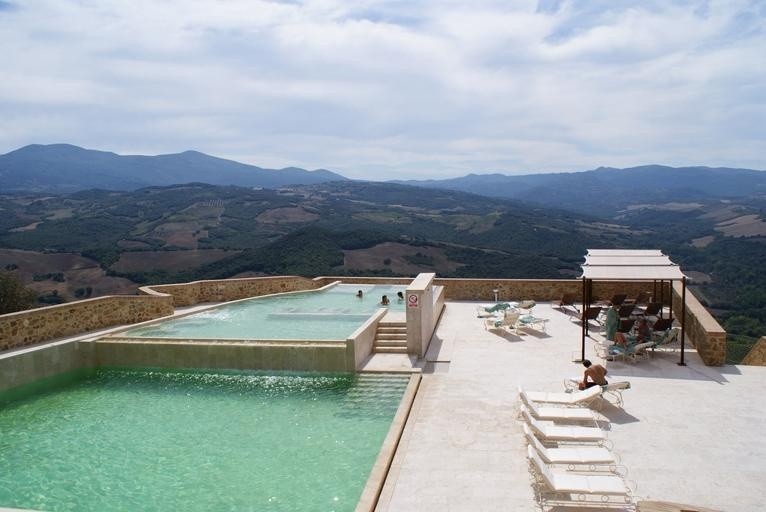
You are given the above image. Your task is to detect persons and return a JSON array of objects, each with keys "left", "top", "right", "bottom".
[
  {"left": 378, "top": 295, "right": 390, "bottom": 305},
  {"left": 356, "top": 289, "right": 363, "bottom": 298},
  {"left": 396, "top": 292, "right": 405, "bottom": 304},
  {"left": 607, "top": 302, "right": 650, "bottom": 344},
  {"left": 579, "top": 359, "right": 609, "bottom": 390}
]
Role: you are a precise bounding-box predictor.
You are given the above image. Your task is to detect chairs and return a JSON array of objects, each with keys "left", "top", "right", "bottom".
[
  {"left": 517, "top": 402, "right": 613, "bottom": 445},
  {"left": 524, "top": 379, "right": 603, "bottom": 405},
  {"left": 521, "top": 420, "right": 623, "bottom": 471},
  {"left": 477, "top": 286, "right": 684, "bottom": 367},
  {"left": 517, "top": 383, "right": 602, "bottom": 426},
  {"left": 524, "top": 444, "right": 640, "bottom": 512},
  {"left": 563, "top": 370, "right": 632, "bottom": 408}
]
